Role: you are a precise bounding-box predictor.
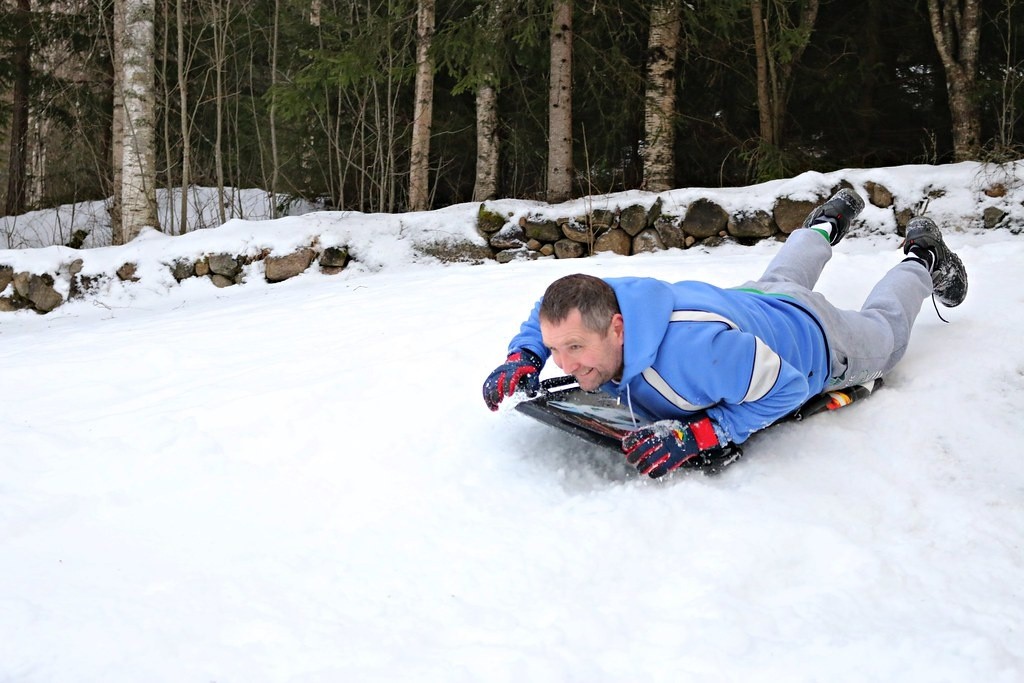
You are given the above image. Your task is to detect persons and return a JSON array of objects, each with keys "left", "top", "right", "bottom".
[{"left": 482, "top": 183, "right": 969, "bottom": 480}]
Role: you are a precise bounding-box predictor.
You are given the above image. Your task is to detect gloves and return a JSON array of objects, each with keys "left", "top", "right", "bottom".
[
  {"left": 622, "top": 409, "right": 723, "bottom": 479},
  {"left": 483, "top": 348, "right": 543, "bottom": 412}
]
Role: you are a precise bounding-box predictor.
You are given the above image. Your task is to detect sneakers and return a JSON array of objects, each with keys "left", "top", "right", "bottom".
[
  {"left": 897, "top": 216, "right": 968, "bottom": 323},
  {"left": 802, "top": 188, "right": 865, "bottom": 246}
]
